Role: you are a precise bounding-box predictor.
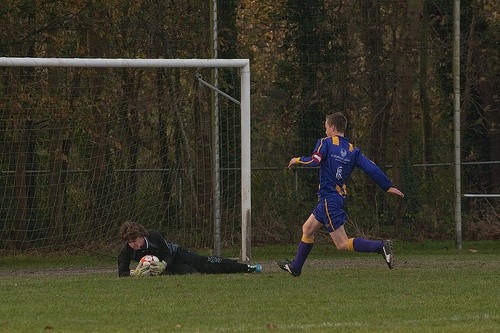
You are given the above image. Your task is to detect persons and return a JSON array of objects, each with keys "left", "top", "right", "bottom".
[
  {"left": 277, "top": 113, "right": 405, "bottom": 278},
  {"left": 118, "top": 221, "right": 263, "bottom": 277}
]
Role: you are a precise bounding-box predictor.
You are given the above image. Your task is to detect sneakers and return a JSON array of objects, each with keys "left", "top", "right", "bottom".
[
  {"left": 277, "top": 260, "right": 302, "bottom": 277},
  {"left": 380, "top": 238, "right": 394, "bottom": 270},
  {"left": 248, "top": 263, "right": 263, "bottom": 273}
]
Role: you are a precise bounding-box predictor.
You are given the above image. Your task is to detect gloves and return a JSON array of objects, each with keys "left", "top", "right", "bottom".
[
  {"left": 151, "top": 260, "right": 166, "bottom": 275},
  {"left": 130, "top": 264, "right": 149, "bottom": 279}
]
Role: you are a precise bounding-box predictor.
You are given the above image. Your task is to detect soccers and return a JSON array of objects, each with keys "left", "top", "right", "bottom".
[{"left": 140, "top": 255, "right": 159, "bottom": 266}]
